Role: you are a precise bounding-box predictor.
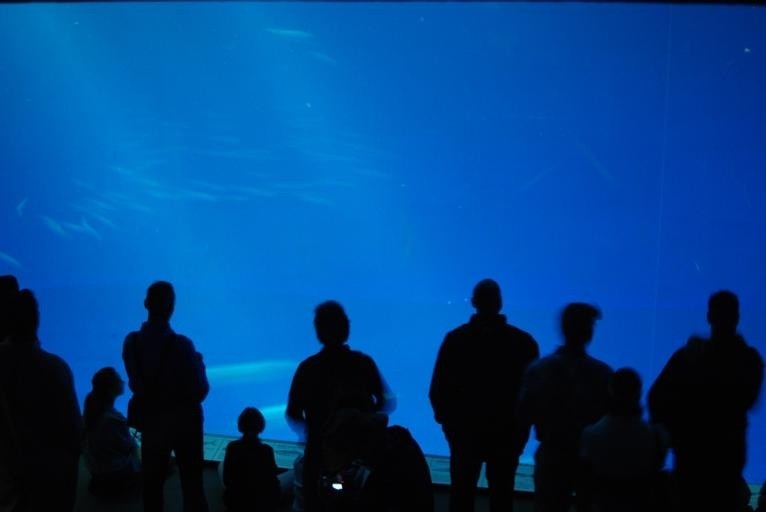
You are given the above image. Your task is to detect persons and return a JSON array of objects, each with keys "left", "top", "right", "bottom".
[{"left": 1, "top": 273, "right": 766, "bottom": 512}]
